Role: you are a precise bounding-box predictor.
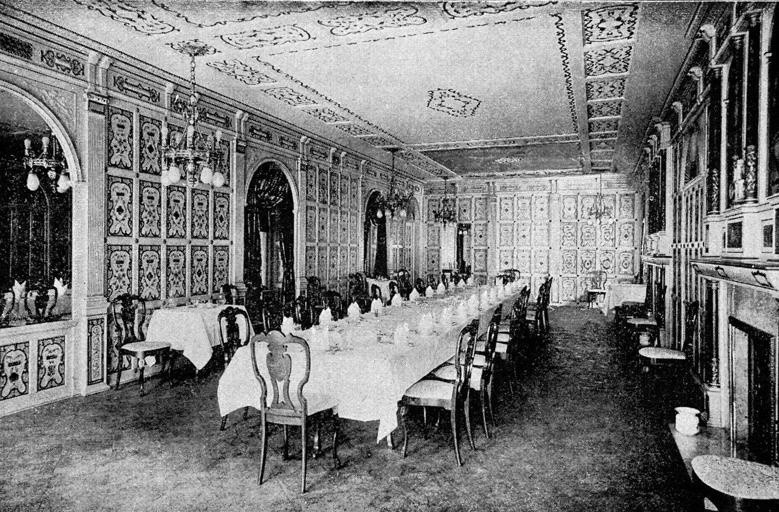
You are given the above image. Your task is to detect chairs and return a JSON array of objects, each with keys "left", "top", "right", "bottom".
[
  {"left": 1, "top": 276, "right": 71, "bottom": 328},
  {"left": 584, "top": 270, "right": 608, "bottom": 310}
]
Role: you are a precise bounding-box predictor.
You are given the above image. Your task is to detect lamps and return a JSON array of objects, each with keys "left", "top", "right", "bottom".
[
  {"left": 160, "top": 40, "right": 227, "bottom": 188},
  {"left": 22, "top": 136, "right": 73, "bottom": 192},
  {"left": 588, "top": 172, "right": 614, "bottom": 226},
  {"left": 432, "top": 177, "right": 457, "bottom": 229},
  {"left": 376, "top": 147, "right": 414, "bottom": 219}
]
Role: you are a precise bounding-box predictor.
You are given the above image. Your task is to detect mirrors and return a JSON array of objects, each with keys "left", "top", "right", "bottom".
[{"left": 0, "top": 86, "right": 72, "bottom": 327}]
